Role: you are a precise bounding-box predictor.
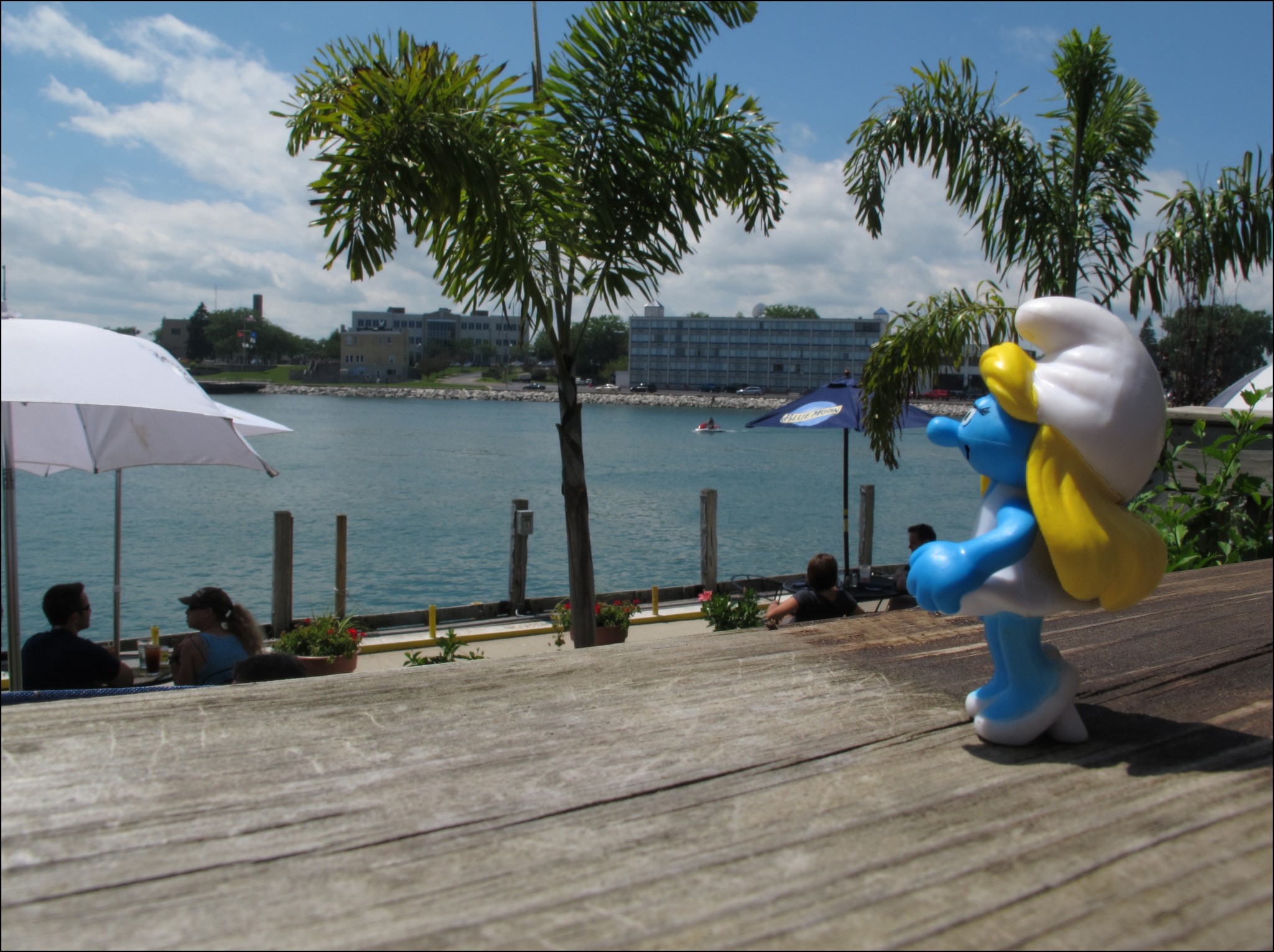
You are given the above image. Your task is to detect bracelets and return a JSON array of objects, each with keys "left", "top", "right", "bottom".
[{"left": 168, "top": 655, "right": 179, "bottom": 663}]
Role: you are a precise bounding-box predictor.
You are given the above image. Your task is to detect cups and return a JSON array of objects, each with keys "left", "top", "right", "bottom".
[
  {"left": 145, "top": 645, "right": 160, "bottom": 673},
  {"left": 136, "top": 638, "right": 154, "bottom": 670},
  {"left": 160, "top": 646, "right": 170, "bottom": 666},
  {"left": 859, "top": 564, "right": 871, "bottom": 583}
]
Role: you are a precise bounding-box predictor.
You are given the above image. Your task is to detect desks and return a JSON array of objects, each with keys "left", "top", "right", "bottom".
[{"left": 783, "top": 577, "right": 909, "bottom": 613}]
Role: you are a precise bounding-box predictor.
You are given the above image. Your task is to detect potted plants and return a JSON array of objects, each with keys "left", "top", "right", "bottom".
[{"left": 296, "top": 647, "right": 361, "bottom": 677}]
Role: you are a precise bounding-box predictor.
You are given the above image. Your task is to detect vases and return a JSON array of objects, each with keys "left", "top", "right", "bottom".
[{"left": 569, "top": 627, "right": 628, "bottom": 648}]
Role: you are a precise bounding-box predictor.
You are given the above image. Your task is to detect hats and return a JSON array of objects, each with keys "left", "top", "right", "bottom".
[{"left": 179, "top": 587, "right": 233, "bottom": 610}]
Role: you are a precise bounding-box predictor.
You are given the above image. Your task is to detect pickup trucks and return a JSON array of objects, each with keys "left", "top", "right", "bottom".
[{"left": 699, "top": 383, "right": 721, "bottom": 393}]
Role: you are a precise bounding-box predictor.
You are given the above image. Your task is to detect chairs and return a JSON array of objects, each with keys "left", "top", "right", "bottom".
[{"left": 731, "top": 574, "right": 804, "bottom": 626}]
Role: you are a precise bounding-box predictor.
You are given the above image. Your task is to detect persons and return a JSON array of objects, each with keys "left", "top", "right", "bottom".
[
  {"left": 21, "top": 582, "right": 135, "bottom": 691},
  {"left": 907, "top": 524, "right": 937, "bottom": 553},
  {"left": 169, "top": 587, "right": 264, "bottom": 685},
  {"left": 766, "top": 554, "right": 866, "bottom": 625}
]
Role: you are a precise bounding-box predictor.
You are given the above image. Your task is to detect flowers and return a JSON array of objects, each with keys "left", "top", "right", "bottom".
[
  {"left": 552, "top": 592, "right": 643, "bottom": 646},
  {"left": 697, "top": 588, "right": 760, "bottom": 632},
  {"left": 275, "top": 600, "right": 372, "bottom": 664}
]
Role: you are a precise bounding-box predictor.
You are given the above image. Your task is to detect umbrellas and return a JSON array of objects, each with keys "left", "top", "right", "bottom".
[
  {"left": 1, "top": 300, "right": 279, "bottom": 689},
  {"left": 113, "top": 401, "right": 294, "bottom": 659},
  {"left": 745, "top": 369, "right": 936, "bottom": 575}
]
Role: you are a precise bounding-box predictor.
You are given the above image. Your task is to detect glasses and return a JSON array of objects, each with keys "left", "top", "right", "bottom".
[{"left": 81, "top": 604, "right": 90, "bottom": 611}]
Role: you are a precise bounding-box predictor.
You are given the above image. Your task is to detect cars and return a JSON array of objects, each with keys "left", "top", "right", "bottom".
[
  {"left": 523, "top": 383, "right": 545, "bottom": 390},
  {"left": 595, "top": 384, "right": 621, "bottom": 393},
  {"left": 576, "top": 378, "right": 593, "bottom": 385}
]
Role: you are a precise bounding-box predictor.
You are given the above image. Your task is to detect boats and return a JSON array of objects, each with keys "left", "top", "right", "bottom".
[{"left": 695, "top": 424, "right": 726, "bottom": 433}]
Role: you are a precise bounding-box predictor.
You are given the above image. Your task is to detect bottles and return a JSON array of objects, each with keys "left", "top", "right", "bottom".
[
  {"left": 852, "top": 573, "right": 858, "bottom": 587},
  {"left": 151, "top": 625, "right": 160, "bottom": 647},
  {"left": 846, "top": 576, "right": 851, "bottom": 588}
]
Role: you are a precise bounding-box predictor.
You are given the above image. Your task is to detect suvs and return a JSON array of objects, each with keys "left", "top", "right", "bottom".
[
  {"left": 736, "top": 386, "right": 763, "bottom": 397},
  {"left": 630, "top": 383, "right": 657, "bottom": 392},
  {"left": 924, "top": 389, "right": 949, "bottom": 401}
]
